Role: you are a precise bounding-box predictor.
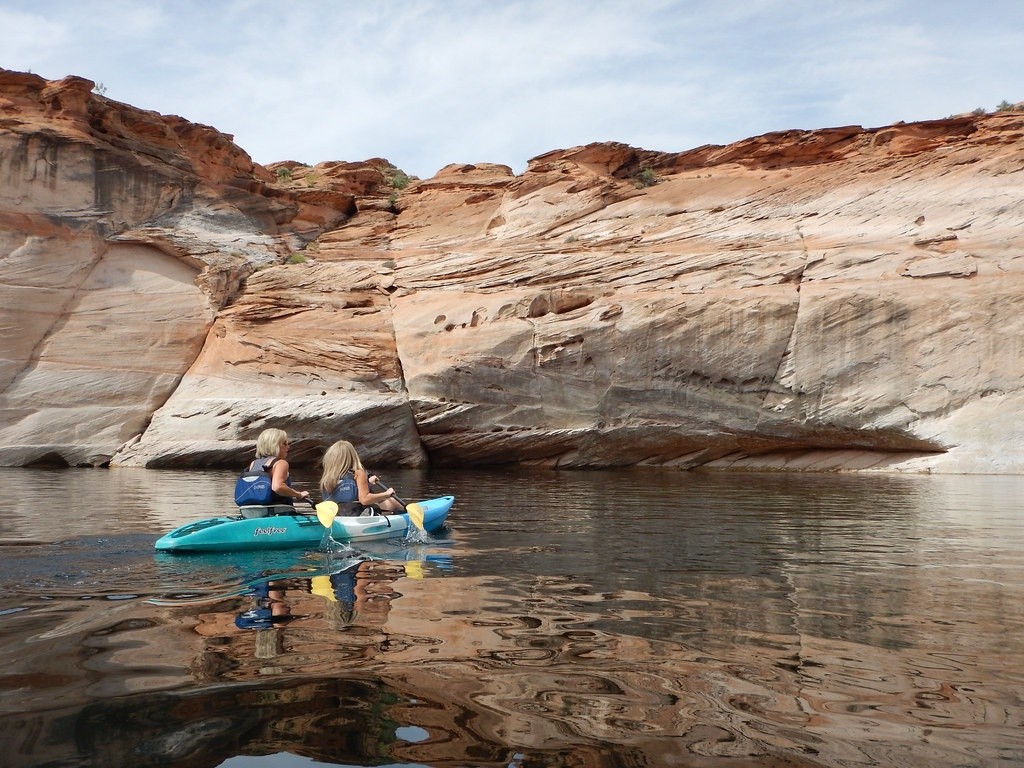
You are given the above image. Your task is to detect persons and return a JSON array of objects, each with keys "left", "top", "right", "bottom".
[
  {"left": 238, "top": 427, "right": 310, "bottom": 519},
  {"left": 318, "top": 440, "right": 407, "bottom": 516},
  {"left": 307, "top": 551, "right": 374, "bottom": 624},
  {"left": 234, "top": 567, "right": 294, "bottom": 632}
]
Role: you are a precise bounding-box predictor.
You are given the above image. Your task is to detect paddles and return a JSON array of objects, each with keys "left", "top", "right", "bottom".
[
  {"left": 364, "top": 469, "right": 424, "bottom": 532},
  {"left": 303, "top": 496, "right": 338, "bottom": 528}
]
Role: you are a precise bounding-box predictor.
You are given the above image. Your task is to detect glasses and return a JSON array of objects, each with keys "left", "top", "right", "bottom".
[{"left": 282, "top": 441, "right": 288, "bottom": 446}]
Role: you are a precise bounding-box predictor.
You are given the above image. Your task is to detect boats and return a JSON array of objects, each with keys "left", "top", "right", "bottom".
[{"left": 155, "top": 495, "right": 458, "bottom": 552}]
